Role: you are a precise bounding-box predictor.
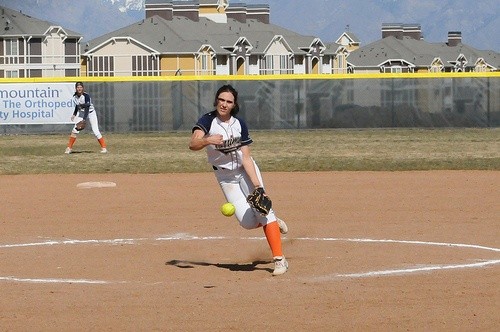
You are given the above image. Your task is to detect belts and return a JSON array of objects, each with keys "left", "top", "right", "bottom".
[{"left": 213, "top": 166, "right": 225, "bottom": 170}]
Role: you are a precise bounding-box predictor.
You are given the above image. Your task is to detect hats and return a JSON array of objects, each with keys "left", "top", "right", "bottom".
[{"left": 76, "top": 82, "right": 83, "bottom": 87}]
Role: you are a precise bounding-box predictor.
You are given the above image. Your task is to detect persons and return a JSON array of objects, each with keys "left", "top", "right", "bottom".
[
  {"left": 189, "top": 85, "right": 288, "bottom": 276},
  {"left": 64, "top": 82, "right": 107, "bottom": 154}
]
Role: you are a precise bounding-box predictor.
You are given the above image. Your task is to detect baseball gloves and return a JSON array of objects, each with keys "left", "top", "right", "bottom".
[
  {"left": 75, "top": 120, "right": 87, "bottom": 131},
  {"left": 246, "top": 187, "right": 273, "bottom": 217}
]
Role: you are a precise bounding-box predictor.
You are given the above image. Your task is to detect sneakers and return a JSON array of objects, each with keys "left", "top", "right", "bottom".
[
  {"left": 65, "top": 147, "right": 72, "bottom": 155},
  {"left": 100, "top": 148, "right": 107, "bottom": 153},
  {"left": 272, "top": 255, "right": 288, "bottom": 276},
  {"left": 275, "top": 217, "right": 288, "bottom": 234}
]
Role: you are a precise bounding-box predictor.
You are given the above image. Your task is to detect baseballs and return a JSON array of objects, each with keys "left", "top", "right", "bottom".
[{"left": 221, "top": 202, "right": 236, "bottom": 218}]
